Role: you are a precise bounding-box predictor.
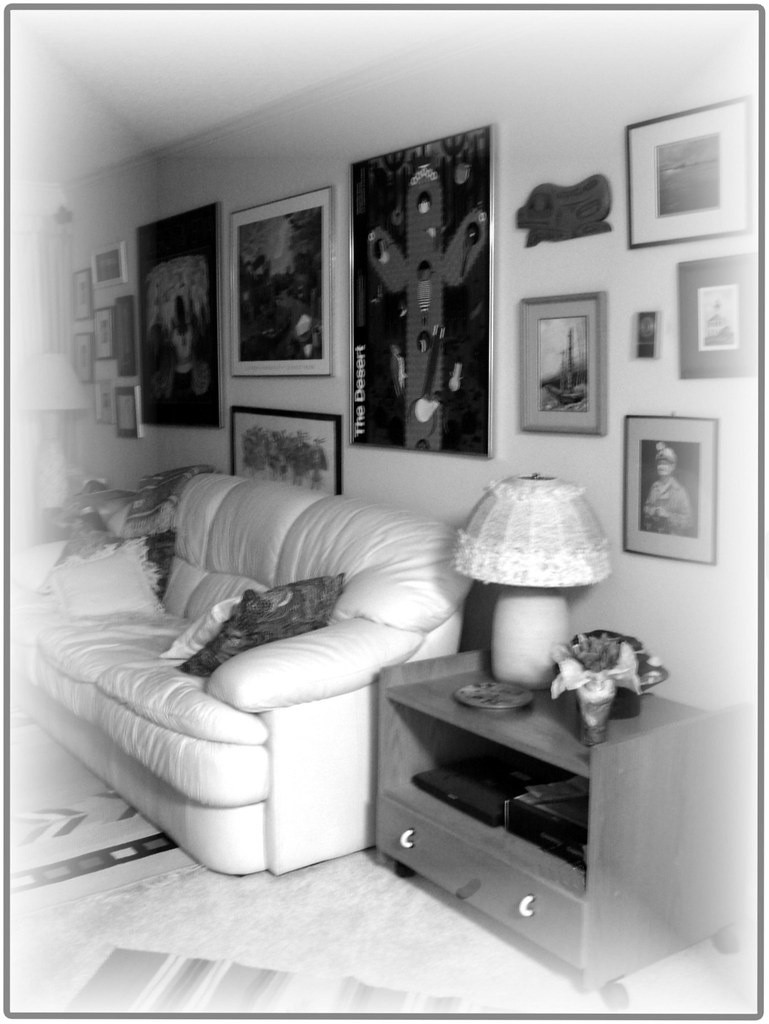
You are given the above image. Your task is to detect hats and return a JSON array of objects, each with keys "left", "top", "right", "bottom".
[{"left": 655, "top": 448, "right": 679, "bottom": 465}]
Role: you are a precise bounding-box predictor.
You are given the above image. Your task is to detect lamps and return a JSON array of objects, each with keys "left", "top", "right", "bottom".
[{"left": 455, "top": 474, "right": 612, "bottom": 689}]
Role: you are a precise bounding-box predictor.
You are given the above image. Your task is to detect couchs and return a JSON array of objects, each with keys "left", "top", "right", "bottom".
[{"left": 27, "top": 474, "right": 473, "bottom": 877}]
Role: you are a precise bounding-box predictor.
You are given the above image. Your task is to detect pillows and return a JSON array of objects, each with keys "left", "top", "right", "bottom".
[
  {"left": 51, "top": 536, "right": 166, "bottom": 616},
  {"left": 176, "top": 572, "right": 345, "bottom": 675},
  {"left": 161, "top": 596, "right": 244, "bottom": 658}
]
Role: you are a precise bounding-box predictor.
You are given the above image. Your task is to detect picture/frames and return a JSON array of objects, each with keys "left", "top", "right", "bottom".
[
  {"left": 626, "top": 95, "right": 751, "bottom": 248},
  {"left": 227, "top": 185, "right": 332, "bottom": 377},
  {"left": 70, "top": 242, "right": 136, "bottom": 439},
  {"left": 623, "top": 415, "right": 718, "bottom": 565},
  {"left": 230, "top": 405, "right": 341, "bottom": 496},
  {"left": 519, "top": 291, "right": 608, "bottom": 434}
]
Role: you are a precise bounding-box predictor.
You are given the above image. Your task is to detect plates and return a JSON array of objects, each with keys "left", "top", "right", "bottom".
[{"left": 452, "top": 681, "right": 535, "bottom": 710}]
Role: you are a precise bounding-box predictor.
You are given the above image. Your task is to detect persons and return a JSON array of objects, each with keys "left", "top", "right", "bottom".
[
  {"left": 643, "top": 448, "right": 696, "bottom": 536},
  {"left": 142, "top": 281, "right": 211, "bottom": 397}
]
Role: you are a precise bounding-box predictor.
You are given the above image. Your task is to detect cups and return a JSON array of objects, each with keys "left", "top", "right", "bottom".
[{"left": 576, "top": 676, "right": 616, "bottom": 746}]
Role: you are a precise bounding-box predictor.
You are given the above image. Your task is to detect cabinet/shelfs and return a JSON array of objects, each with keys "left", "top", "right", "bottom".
[{"left": 373, "top": 645, "right": 707, "bottom": 983}]
